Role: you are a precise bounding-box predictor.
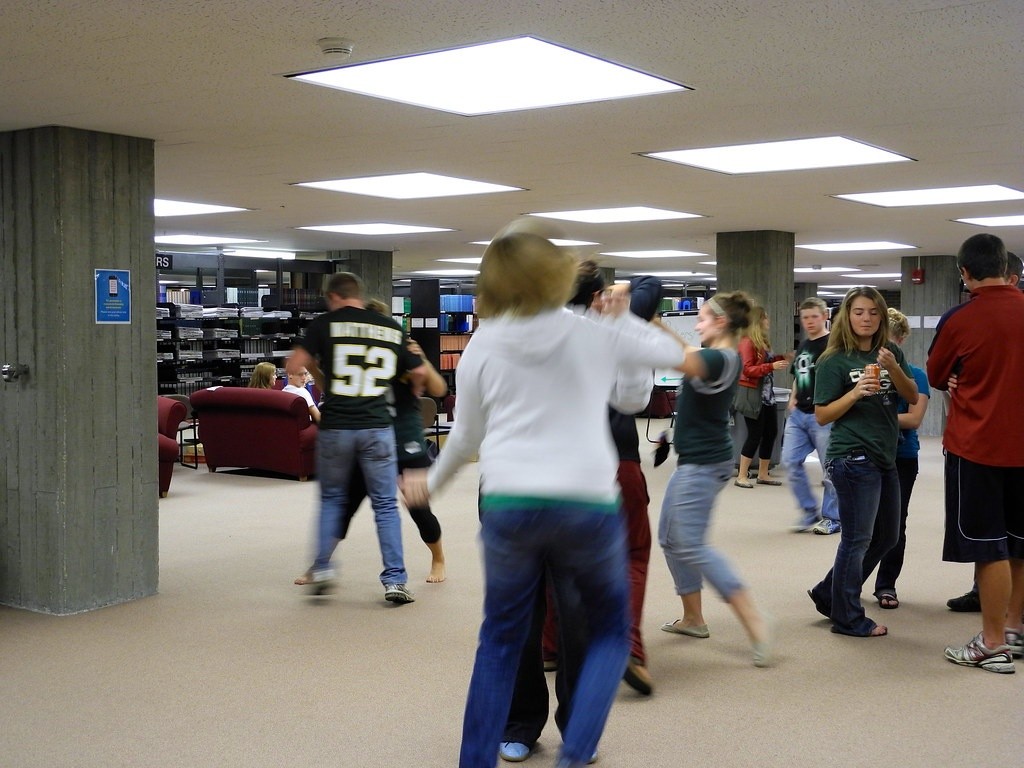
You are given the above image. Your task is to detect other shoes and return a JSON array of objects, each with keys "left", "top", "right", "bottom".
[
  {"left": 621, "top": 654, "right": 657, "bottom": 697},
  {"left": 499, "top": 741, "right": 529, "bottom": 763},
  {"left": 383, "top": 583, "right": 415, "bottom": 604},
  {"left": 542, "top": 652, "right": 558, "bottom": 672},
  {"left": 753, "top": 639, "right": 787, "bottom": 668},
  {"left": 733, "top": 479, "right": 753, "bottom": 488},
  {"left": 755, "top": 477, "right": 783, "bottom": 486},
  {"left": 947, "top": 590, "right": 984, "bottom": 614},
  {"left": 662, "top": 617, "right": 713, "bottom": 639}
]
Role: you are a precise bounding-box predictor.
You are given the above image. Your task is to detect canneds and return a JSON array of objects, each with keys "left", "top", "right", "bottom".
[{"left": 865, "top": 363, "right": 881, "bottom": 393}]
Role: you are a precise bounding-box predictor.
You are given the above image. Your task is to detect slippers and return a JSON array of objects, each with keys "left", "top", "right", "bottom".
[
  {"left": 806, "top": 589, "right": 834, "bottom": 618},
  {"left": 830, "top": 623, "right": 888, "bottom": 638}
]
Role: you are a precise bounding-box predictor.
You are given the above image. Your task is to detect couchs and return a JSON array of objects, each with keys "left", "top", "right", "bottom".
[
  {"left": 157, "top": 397, "right": 187, "bottom": 499},
  {"left": 189, "top": 386, "right": 318, "bottom": 482}
]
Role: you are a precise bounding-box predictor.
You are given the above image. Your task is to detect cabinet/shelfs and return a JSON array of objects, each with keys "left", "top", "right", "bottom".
[
  {"left": 630, "top": 276, "right": 716, "bottom": 316},
  {"left": 157, "top": 249, "right": 353, "bottom": 391},
  {"left": 391, "top": 278, "right": 483, "bottom": 406}
]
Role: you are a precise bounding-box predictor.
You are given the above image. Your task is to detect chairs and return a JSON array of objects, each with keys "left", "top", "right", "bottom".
[
  {"left": 416, "top": 396, "right": 440, "bottom": 452},
  {"left": 164, "top": 394, "right": 200, "bottom": 470}
]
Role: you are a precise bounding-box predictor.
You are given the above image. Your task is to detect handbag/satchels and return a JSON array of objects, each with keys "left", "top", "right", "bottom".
[{"left": 733, "top": 385, "right": 762, "bottom": 420}]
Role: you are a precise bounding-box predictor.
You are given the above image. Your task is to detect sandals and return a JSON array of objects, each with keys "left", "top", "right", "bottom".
[{"left": 877, "top": 593, "right": 900, "bottom": 609}]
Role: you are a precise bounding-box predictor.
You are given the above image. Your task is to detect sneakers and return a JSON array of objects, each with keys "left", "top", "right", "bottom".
[
  {"left": 790, "top": 514, "right": 823, "bottom": 532},
  {"left": 942, "top": 632, "right": 1017, "bottom": 674},
  {"left": 1004, "top": 626, "right": 1024, "bottom": 657},
  {"left": 814, "top": 517, "right": 842, "bottom": 535}
]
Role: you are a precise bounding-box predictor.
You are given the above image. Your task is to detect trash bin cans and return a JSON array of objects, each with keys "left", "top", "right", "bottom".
[{"left": 732, "top": 387, "right": 792, "bottom": 478}]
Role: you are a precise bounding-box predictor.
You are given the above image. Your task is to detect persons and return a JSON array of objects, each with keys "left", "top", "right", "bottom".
[
  {"left": 404, "top": 232, "right": 774, "bottom": 768},
  {"left": 807, "top": 287, "right": 919, "bottom": 637},
  {"left": 926, "top": 233, "right": 1024, "bottom": 672},
  {"left": 733, "top": 306, "right": 797, "bottom": 489},
  {"left": 247, "top": 362, "right": 278, "bottom": 389},
  {"left": 781, "top": 296, "right": 842, "bottom": 535},
  {"left": 281, "top": 362, "right": 320, "bottom": 423},
  {"left": 328, "top": 298, "right": 447, "bottom": 582},
  {"left": 285, "top": 271, "right": 427, "bottom": 601},
  {"left": 874, "top": 305, "right": 930, "bottom": 608}
]
